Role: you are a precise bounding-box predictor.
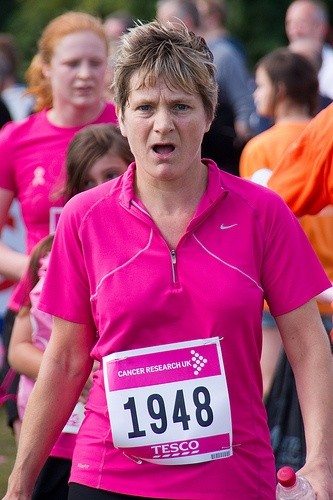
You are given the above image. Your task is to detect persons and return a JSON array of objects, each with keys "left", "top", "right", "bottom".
[
  {"left": 232, "top": 37, "right": 332, "bottom": 147},
  {"left": 284, "top": 0, "right": 333, "bottom": 99},
  {"left": 1, "top": 15, "right": 333, "bottom": 500},
  {"left": 238, "top": 49, "right": 322, "bottom": 192},
  {"left": 0, "top": 40, "right": 36, "bottom": 442},
  {"left": 7, "top": 120, "right": 135, "bottom": 500},
  {"left": 0, "top": 9, "right": 121, "bottom": 279},
  {"left": 240, "top": 99, "right": 332, "bottom": 484},
  {"left": 97, "top": 0, "right": 272, "bottom": 179}
]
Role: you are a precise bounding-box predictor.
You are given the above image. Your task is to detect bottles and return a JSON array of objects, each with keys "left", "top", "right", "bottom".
[{"left": 276, "top": 466, "right": 317, "bottom": 500}]
